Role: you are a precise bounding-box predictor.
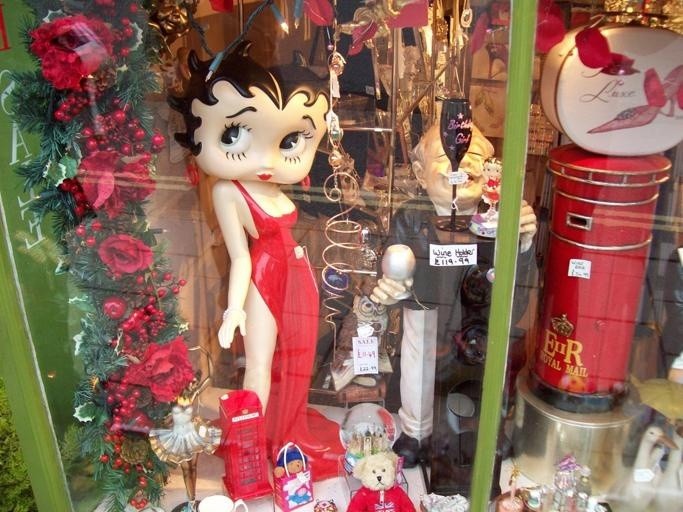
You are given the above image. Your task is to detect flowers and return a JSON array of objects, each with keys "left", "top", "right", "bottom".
[{"left": 25, "top": 3, "right": 195, "bottom": 419}]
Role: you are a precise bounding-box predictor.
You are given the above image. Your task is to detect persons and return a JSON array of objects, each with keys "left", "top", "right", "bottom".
[
  {"left": 368, "top": 119, "right": 537, "bottom": 500},
  {"left": 166, "top": 41, "right": 344, "bottom": 483},
  {"left": 146, "top": 1, "right": 191, "bottom": 99},
  {"left": 122, "top": 346, "right": 222, "bottom": 512},
  {"left": 482, "top": 157, "right": 503, "bottom": 217}
]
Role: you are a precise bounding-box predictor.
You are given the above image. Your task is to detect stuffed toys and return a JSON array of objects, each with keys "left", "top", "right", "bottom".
[
  {"left": 348, "top": 452, "right": 417, "bottom": 512},
  {"left": 274, "top": 449, "right": 308, "bottom": 478}
]
